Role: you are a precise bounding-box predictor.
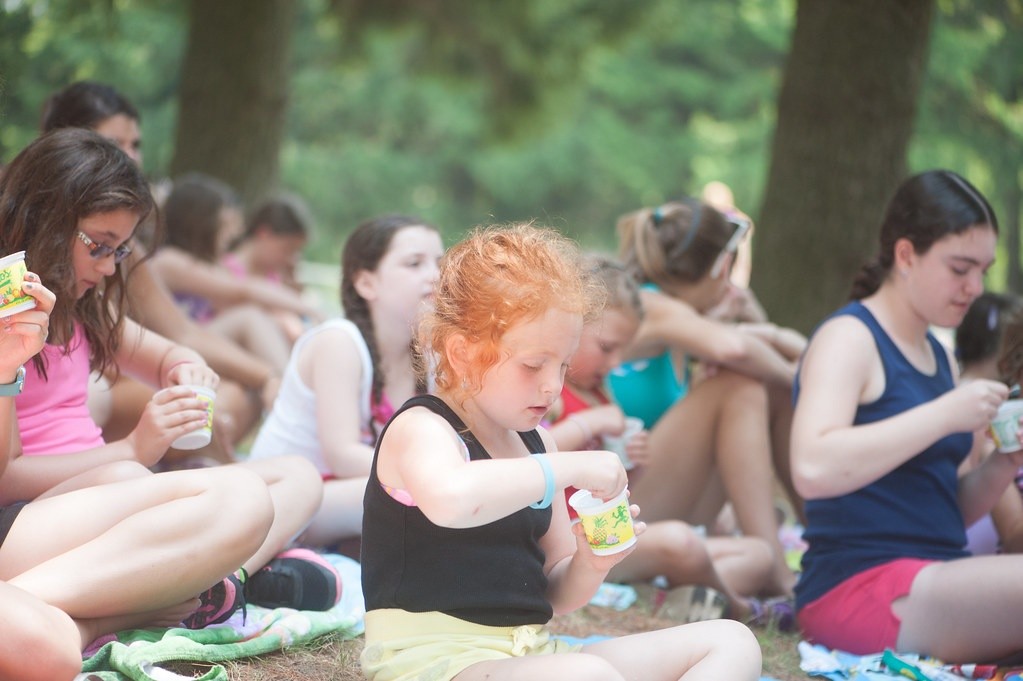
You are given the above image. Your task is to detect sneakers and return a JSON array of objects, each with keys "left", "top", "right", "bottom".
[
  {"left": 242, "top": 548, "right": 342, "bottom": 613},
  {"left": 182, "top": 572, "right": 245, "bottom": 629}
]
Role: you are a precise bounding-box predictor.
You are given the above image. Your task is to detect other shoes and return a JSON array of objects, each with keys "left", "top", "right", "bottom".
[
  {"left": 742, "top": 597, "right": 795, "bottom": 633},
  {"left": 620, "top": 579, "right": 728, "bottom": 628}
]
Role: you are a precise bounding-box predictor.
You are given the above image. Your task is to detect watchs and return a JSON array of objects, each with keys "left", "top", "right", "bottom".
[{"left": 0, "top": 367, "right": 26, "bottom": 397}]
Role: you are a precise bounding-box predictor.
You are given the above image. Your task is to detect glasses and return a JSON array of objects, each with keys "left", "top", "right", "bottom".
[
  {"left": 77, "top": 231, "right": 132, "bottom": 264},
  {"left": 709, "top": 218, "right": 753, "bottom": 281}
]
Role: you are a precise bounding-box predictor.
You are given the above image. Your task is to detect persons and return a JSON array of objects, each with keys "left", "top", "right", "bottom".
[
  {"left": 0, "top": 271, "right": 274, "bottom": 681},
  {"left": 39, "top": 80, "right": 311, "bottom": 466},
  {"left": 542, "top": 201, "right": 808, "bottom": 628},
  {"left": 956, "top": 292, "right": 1023, "bottom": 556},
  {"left": 249, "top": 218, "right": 445, "bottom": 547},
  {"left": 0, "top": 125, "right": 342, "bottom": 630},
  {"left": 359, "top": 228, "right": 763, "bottom": 681},
  {"left": 790, "top": 171, "right": 1023, "bottom": 664}
]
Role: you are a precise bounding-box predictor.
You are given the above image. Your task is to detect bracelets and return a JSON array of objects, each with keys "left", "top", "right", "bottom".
[
  {"left": 170, "top": 360, "right": 192, "bottom": 371},
  {"left": 529, "top": 454, "right": 555, "bottom": 509}
]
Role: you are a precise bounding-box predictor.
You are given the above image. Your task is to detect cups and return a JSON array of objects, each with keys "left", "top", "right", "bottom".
[
  {"left": 0, "top": 248, "right": 37, "bottom": 319},
  {"left": 601, "top": 416, "right": 645, "bottom": 471},
  {"left": 153, "top": 385, "right": 216, "bottom": 451},
  {"left": 568, "top": 484, "right": 638, "bottom": 556},
  {"left": 990, "top": 399, "right": 1023, "bottom": 454}
]
